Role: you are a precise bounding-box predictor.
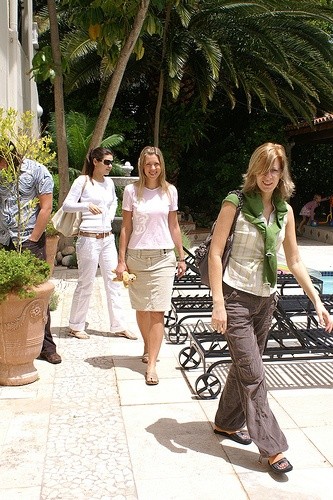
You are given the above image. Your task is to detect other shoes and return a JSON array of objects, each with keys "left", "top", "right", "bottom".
[
  {"left": 42, "top": 351, "right": 62, "bottom": 364},
  {"left": 115, "top": 330, "right": 138, "bottom": 340},
  {"left": 141, "top": 352, "right": 149, "bottom": 363},
  {"left": 145, "top": 369, "right": 159, "bottom": 385},
  {"left": 65, "top": 329, "right": 90, "bottom": 340}
]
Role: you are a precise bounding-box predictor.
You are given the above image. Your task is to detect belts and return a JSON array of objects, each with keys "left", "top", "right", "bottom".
[{"left": 78, "top": 232, "right": 112, "bottom": 239}]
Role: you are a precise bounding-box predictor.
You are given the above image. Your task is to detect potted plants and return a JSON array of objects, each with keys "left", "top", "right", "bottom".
[{"left": 0, "top": 105, "right": 56, "bottom": 386}]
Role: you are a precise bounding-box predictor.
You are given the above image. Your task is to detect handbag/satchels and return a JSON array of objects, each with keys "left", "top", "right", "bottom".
[
  {"left": 195, "top": 190, "right": 244, "bottom": 287},
  {"left": 50, "top": 175, "right": 89, "bottom": 239}
]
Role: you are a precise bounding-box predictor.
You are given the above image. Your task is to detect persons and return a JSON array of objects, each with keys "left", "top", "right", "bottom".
[
  {"left": 116, "top": 146, "right": 185, "bottom": 385},
  {"left": 209, "top": 143, "right": 333, "bottom": 472},
  {"left": 62, "top": 147, "right": 138, "bottom": 339},
  {"left": 296, "top": 194, "right": 329, "bottom": 234},
  {"left": 0, "top": 142, "right": 62, "bottom": 363}
]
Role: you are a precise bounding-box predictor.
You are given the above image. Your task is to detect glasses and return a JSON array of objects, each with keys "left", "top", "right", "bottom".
[{"left": 96, "top": 159, "right": 115, "bottom": 166}]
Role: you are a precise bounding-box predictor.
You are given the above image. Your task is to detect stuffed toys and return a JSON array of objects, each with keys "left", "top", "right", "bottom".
[{"left": 112, "top": 269, "right": 136, "bottom": 288}]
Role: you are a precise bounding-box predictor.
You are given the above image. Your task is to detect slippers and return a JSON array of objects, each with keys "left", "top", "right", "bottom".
[
  {"left": 213, "top": 426, "right": 252, "bottom": 445},
  {"left": 268, "top": 454, "right": 293, "bottom": 473}
]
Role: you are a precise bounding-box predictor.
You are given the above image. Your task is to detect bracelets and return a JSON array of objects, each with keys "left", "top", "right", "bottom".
[{"left": 179, "top": 259, "right": 184, "bottom": 262}]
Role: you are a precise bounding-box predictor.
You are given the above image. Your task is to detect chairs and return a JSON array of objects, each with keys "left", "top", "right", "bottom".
[{"left": 163, "top": 245, "right": 333, "bottom": 400}]
